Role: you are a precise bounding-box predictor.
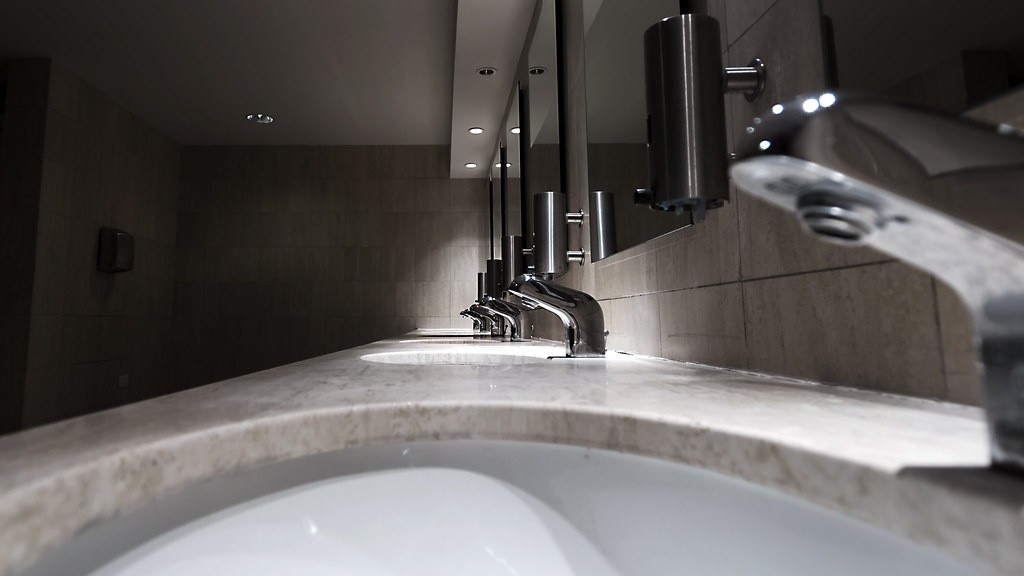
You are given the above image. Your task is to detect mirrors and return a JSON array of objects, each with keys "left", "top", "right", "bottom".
[{"left": 482, "top": 0, "right": 1023, "bottom": 283}]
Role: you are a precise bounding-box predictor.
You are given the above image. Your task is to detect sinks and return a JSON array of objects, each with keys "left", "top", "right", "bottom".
[
  {"left": 0, "top": 401, "right": 1023, "bottom": 576},
  {"left": 399, "top": 338, "right": 498, "bottom": 347},
  {"left": 353, "top": 349, "right": 544, "bottom": 367}
]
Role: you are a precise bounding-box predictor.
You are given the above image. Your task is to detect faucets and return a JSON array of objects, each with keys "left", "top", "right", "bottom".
[
  {"left": 467, "top": 304, "right": 505, "bottom": 338},
  {"left": 508, "top": 274, "right": 611, "bottom": 359},
  {"left": 479, "top": 295, "right": 534, "bottom": 341},
  {"left": 460, "top": 310, "right": 480, "bottom": 331},
  {"left": 730, "top": 89, "right": 1024, "bottom": 471},
  {"left": 464, "top": 309, "right": 490, "bottom": 334}
]
